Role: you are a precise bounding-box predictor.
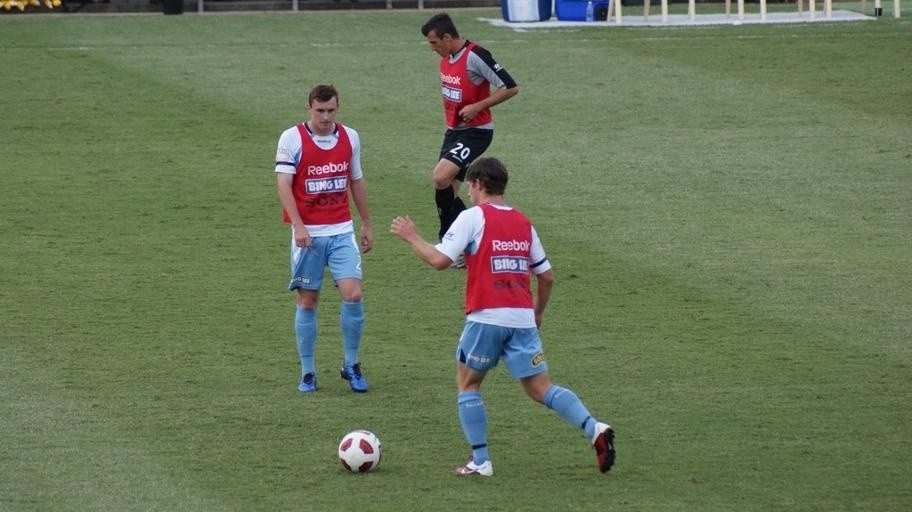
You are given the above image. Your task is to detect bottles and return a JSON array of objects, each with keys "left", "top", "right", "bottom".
[{"left": 585, "top": 1, "right": 594, "bottom": 23}]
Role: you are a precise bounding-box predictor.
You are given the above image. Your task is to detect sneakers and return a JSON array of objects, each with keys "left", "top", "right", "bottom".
[
  {"left": 297, "top": 371, "right": 318, "bottom": 392},
  {"left": 454, "top": 454, "right": 494, "bottom": 476},
  {"left": 341, "top": 360, "right": 368, "bottom": 392},
  {"left": 591, "top": 422, "right": 615, "bottom": 473}
]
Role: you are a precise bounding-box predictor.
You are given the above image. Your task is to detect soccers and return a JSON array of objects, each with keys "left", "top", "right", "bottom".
[{"left": 337, "top": 429, "right": 382, "bottom": 475}]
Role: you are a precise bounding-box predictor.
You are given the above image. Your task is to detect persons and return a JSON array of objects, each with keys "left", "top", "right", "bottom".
[
  {"left": 420, "top": 14, "right": 519, "bottom": 271},
  {"left": 391, "top": 156, "right": 617, "bottom": 477},
  {"left": 273, "top": 84, "right": 375, "bottom": 391}
]
